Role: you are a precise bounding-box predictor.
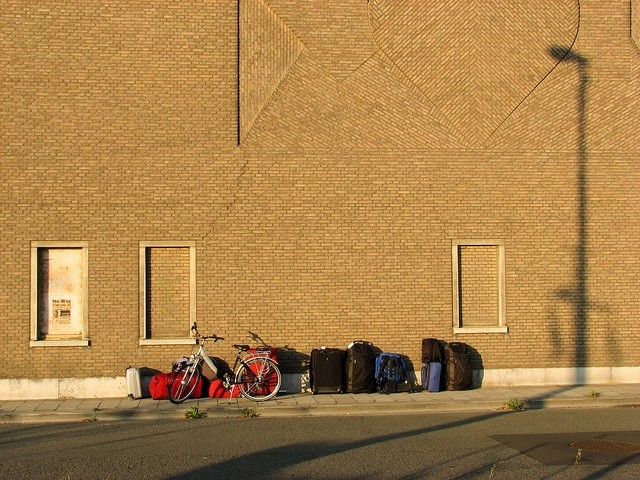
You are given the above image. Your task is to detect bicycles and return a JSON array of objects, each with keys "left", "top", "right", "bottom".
[{"left": 167, "top": 321, "right": 283, "bottom": 405}]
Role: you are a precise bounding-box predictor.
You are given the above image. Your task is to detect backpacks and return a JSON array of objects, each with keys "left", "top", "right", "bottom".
[
  {"left": 347, "top": 340, "right": 377, "bottom": 393},
  {"left": 375, "top": 352, "right": 418, "bottom": 393},
  {"left": 443, "top": 342, "right": 473, "bottom": 390}
]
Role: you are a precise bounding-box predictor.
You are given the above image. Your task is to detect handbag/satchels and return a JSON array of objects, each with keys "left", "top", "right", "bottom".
[
  {"left": 422, "top": 338, "right": 444, "bottom": 362},
  {"left": 210, "top": 378, "right": 244, "bottom": 398},
  {"left": 150, "top": 371, "right": 204, "bottom": 401},
  {"left": 420, "top": 362, "right": 441, "bottom": 392}
]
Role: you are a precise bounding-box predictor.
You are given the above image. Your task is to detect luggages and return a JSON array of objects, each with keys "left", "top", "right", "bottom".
[
  {"left": 310, "top": 348, "right": 344, "bottom": 395},
  {"left": 127, "top": 366, "right": 142, "bottom": 400},
  {"left": 246, "top": 346, "right": 278, "bottom": 397}
]
